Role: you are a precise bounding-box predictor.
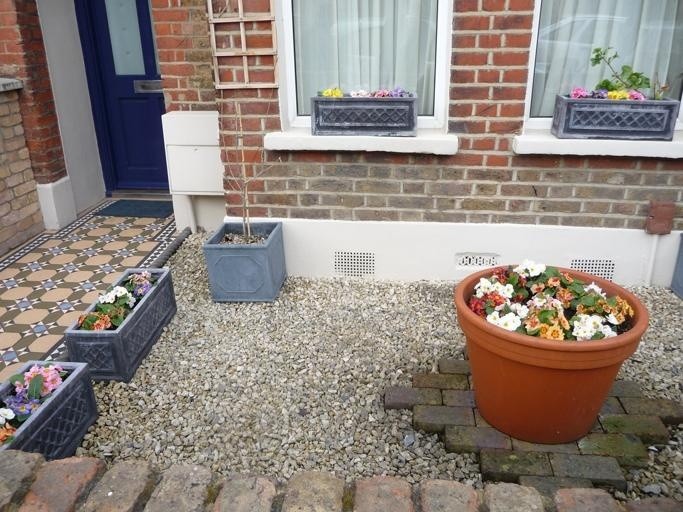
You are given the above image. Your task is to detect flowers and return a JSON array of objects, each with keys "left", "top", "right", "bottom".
[
  {"left": 323, "top": 86, "right": 408, "bottom": 98},
  {"left": 0, "top": 358, "right": 61, "bottom": 442},
  {"left": 470, "top": 261, "right": 634, "bottom": 345},
  {"left": 79, "top": 272, "right": 152, "bottom": 332},
  {"left": 569, "top": 41, "right": 669, "bottom": 103}
]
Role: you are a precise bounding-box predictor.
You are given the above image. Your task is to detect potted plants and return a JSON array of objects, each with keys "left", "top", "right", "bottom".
[{"left": 201, "top": 60, "right": 287, "bottom": 306}]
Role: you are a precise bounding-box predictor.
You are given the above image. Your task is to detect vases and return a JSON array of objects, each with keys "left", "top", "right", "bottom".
[
  {"left": 453, "top": 266, "right": 648, "bottom": 445},
  {"left": 1, "top": 356, "right": 99, "bottom": 461},
  {"left": 311, "top": 89, "right": 417, "bottom": 136},
  {"left": 61, "top": 264, "right": 180, "bottom": 383}
]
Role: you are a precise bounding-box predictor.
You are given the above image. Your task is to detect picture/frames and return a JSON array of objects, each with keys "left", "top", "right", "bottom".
[{"left": 548, "top": 93, "right": 679, "bottom": 142}]
